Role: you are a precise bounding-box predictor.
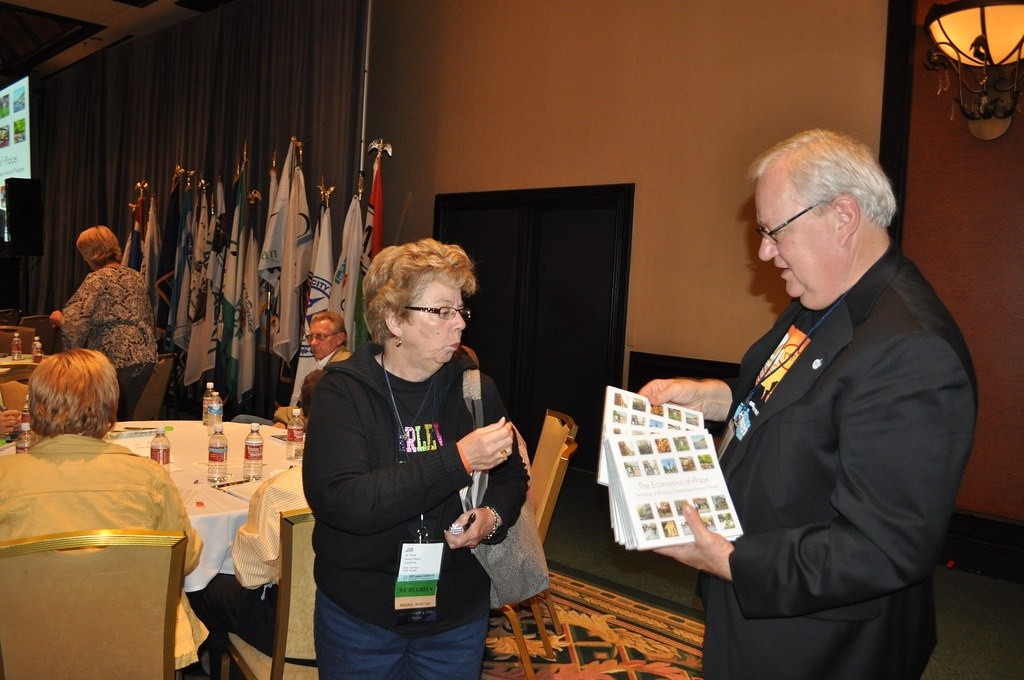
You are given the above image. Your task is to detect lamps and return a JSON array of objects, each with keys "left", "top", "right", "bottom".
[{"left": 924, "top": 0, "right": 1024, "bottom": 141}]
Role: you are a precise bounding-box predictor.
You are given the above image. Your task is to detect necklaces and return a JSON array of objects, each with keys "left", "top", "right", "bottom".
[{"left": 381, "top": 353, "right": 435, "bottom": 440}]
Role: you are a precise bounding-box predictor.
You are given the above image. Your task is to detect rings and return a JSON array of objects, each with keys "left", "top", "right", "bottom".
[{"left": 501, "top": 449, "right": 508, "bottom": 459}]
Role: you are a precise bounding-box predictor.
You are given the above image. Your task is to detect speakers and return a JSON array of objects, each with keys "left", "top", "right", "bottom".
[{"left": 5, "top": 177, "right": 44, "bottom": 257}]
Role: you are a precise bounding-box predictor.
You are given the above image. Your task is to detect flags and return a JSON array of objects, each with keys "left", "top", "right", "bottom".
[{"left": 122, "top": 143, "right": 384, "bottom": 417}]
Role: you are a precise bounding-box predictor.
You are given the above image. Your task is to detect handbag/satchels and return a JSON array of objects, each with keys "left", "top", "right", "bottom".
[{"left": 459, "top": 369, "right": 549, "bottom": 610}]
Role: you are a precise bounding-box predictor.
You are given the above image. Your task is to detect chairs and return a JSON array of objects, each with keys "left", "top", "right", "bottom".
[
  {"left": 485, "top": 411, "right": 579, "bottom": 680},
  {"left": 0, "top": 306, "right": 321, "bottom": 680}
]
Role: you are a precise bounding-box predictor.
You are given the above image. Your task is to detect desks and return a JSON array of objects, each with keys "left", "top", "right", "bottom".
[
  {"left": 1, "top": 421, "right": 304, "bottom": 592},
  {"left": 0, "top": 352, "right": 35, "bottom": 368}
]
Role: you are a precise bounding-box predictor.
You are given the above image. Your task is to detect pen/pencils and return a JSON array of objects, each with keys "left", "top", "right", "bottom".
[{"left": 465, "top": 513, "right": 476, "bottom": 530}]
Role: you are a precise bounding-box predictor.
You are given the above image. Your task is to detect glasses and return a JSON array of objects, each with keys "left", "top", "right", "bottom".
[
  {"left": 404, "top": 306, "right": 471, "bottom": 321},
  {"left": 305, "top": 333, "right": 338, "bottom": 341},
  {"left": 756, "top": 201, "right": 822, "bottom": 245}
]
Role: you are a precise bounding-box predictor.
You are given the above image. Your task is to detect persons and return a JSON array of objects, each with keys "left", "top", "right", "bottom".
[
  {"left": 0, "top": 348, "right": 210, "bottom": 671},
  {"left": 50, "top": 226, "right": 159, "bottom": 421},
  {"left": 202, "top": 370, "right": 325, "bottom": 680},
  {"left": 640, "top": 130, "right": 979, "bottom": 680},
  {"left": 231, "top": 312, "right": 352, "bottom": 431},
  {"left": 0, "top": 410, "right": 23, "bottom": 438},
  {"left": 462, "top": 344, "right": 549, "bottom": 608},
  {"left": 302, "top": 240, "right": 527, "bottom": 680}
]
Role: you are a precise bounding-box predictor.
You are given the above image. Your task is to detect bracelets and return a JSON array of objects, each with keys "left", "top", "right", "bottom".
[{"left": 484, "top": 506, "right": 499, "bottom": 539}]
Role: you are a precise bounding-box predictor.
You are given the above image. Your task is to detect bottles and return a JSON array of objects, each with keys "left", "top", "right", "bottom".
[
  {"left": 207, "top": 425, "right": 227, "bottom": 482},
  {"left": 34, "top": 344, "right": 44, "bottom": 364},
  {"left": 150, "top": 428, "right": 169, "bottom": 474},
  {"left": 242, "top": 422, "right": 263, "bottom": 480},
  {"left": 21, "top": 394, "right": 31, "bottom": 422},
  {"left": 14, "top": 422, "right": 36, "bottom": 452},
  {"left": 11, "top": 333, "right": 22, "bottom": 361},
  {"left": 203, "top": 382, "right": 216, "bottom": 426},
  {"left": 285, "top": 408, "right": 306, "bottom": 462},
  {"left": 208, "top": 391, "right": 223, "bottom": 437},
  {"left": 32, "top": 337, "right": 42, "bottom": 361}
]
